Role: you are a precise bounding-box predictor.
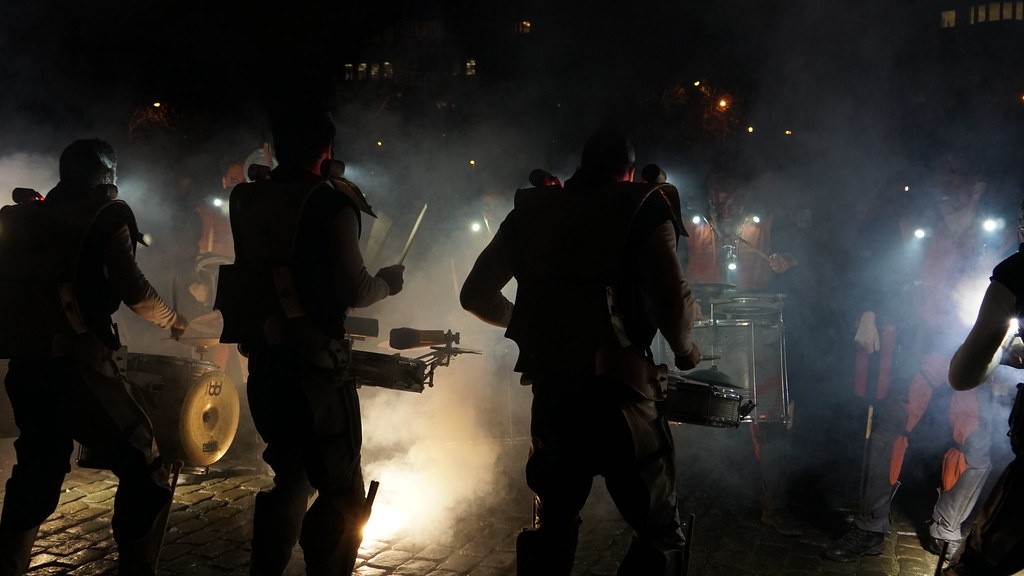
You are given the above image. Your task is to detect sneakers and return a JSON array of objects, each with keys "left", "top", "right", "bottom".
[
  {"left": 824, "top": 525, "right": 886, "bottom": 562},
  {"left": 929, "top": 539, "right": 962, "bottom": 564},
  {"left": 760, "top": 504, "right": 803, "bottom": 537}
]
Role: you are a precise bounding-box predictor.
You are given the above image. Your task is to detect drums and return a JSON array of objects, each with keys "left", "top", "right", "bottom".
[
  {"left": 663, "top": 318, "right": 784, "bottom": 423},
  {"left": 351, "top": 349, "right": 428, "bottom": 393},
  {"left": 664, "top": 375, "right": 744, "bottom": 430},
  {"left": 711, "top": 290, "right": 783, "bottom": 315},
  {"left": 76, "top": 351, "right": 222, "bottom": 476}
]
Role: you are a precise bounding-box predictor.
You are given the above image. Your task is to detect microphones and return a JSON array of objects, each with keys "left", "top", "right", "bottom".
[{"left": 390, "top": 328, "right": 459, "bottom": 349}]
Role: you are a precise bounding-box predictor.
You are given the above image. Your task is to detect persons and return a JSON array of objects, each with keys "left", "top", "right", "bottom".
[
  {"left": 212, "top": 115, "right": 407, "bottom": 576},
  {"left": 683, "top": 140, "right": 1023, "bottom": 576},
  {"left": 127, "top": 90, "right": 340, "bottom": 266},
  {"left": 1, "top": 135, "right": 193, "bottom": 576},
  {"left": 457, "top": 127, "right": 701, "bottom": 576}
]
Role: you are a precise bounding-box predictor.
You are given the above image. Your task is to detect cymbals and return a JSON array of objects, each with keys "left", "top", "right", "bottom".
[
  {"left": 689, "top": 282, "right": 738, "bottom": 300},
  {"left": 160, "top": 337, "right": 220, "bottom": 350},
  {"left": 179, "top": 370, "right": 241, "bottom": 468},
  {"left": 680, "top": 365, "right": 744, "bottom": 389}
]
